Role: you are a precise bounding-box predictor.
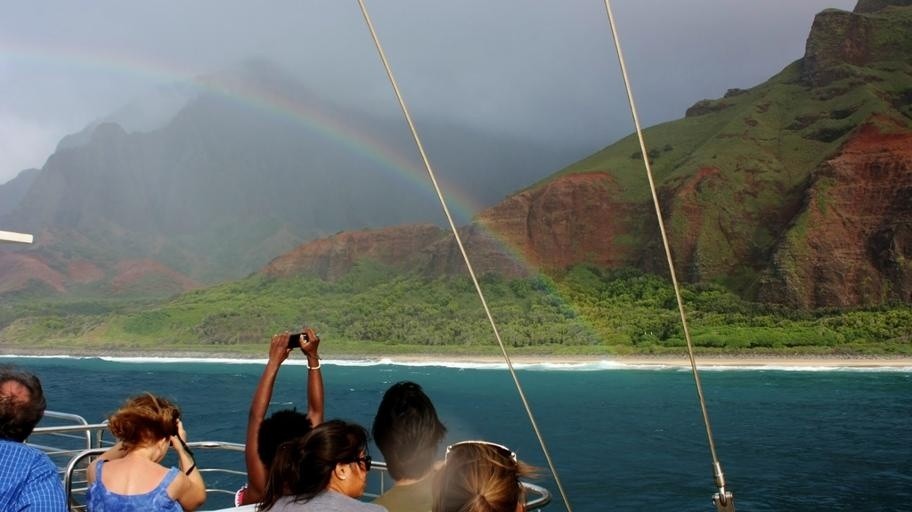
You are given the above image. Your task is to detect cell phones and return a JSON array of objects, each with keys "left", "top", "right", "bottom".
[{"left": 287, "top": 333, "right": 308, "bottom": 348}]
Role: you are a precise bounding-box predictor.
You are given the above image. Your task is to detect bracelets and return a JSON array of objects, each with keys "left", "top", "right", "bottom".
[{"left": 303, "top": 359, "right": 322, "bottom": 370}]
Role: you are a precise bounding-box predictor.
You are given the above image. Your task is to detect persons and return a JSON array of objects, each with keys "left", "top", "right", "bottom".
[
  {"left": 254, "top": 421, "right": 389, "bottom": 511},
  {"left": 0, "top": 362, "right": 72, "bottom": 512},
  {"left": 83, "top": 390, "right": 209, "bottom": 511},
  {"left": 369, "top": 379, "right": 450, "bottom": 511},
  {"left": 431, "top": 440, "right": 551, "bottom": 512},
  {"left": 232, "top": 325, "right": 326, "bottom": 507}
]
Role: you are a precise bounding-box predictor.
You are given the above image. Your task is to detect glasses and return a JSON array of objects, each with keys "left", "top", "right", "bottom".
[
  {"left": 342, "top": 455, "right": 372, "bottom": 471},
  {"left": 444, "top": 438, "right": 519, "bottom": 464}
]
